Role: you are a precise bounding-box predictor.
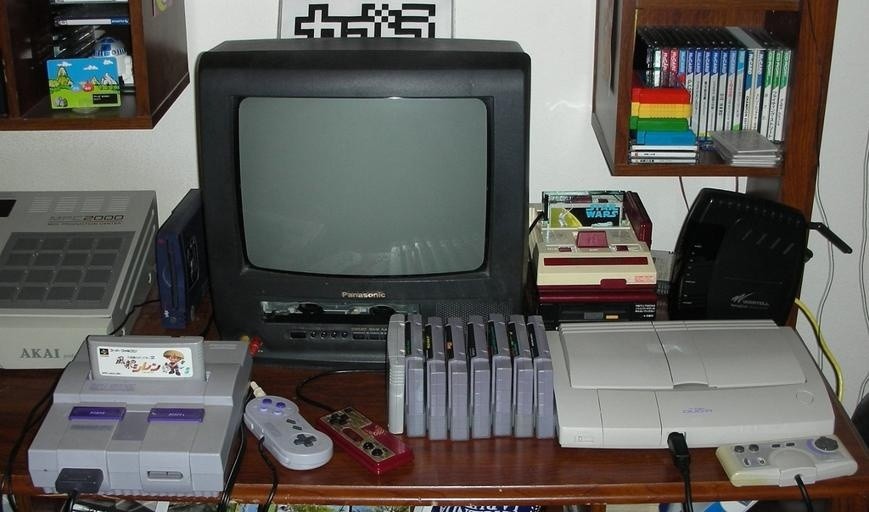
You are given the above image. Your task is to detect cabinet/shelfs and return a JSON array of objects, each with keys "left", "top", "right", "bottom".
[
  {"left": 1, "top": 0, "right": 191, "bottom": 129},
  {"left": 591, "top": 1, "right": 841, "bottom": 325}
]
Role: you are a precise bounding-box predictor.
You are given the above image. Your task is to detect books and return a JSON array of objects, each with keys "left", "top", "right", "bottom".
[
  {"left": 29, "top": 494, "right": 759, "bottom": 512},
  {"left": 626, "top": 25, "right": 793, "bottom": 168}
]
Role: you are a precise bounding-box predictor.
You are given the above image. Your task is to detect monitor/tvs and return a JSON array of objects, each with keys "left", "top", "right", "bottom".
[{"left": 195, "top": 38, "right": 532, "bottom": 372}]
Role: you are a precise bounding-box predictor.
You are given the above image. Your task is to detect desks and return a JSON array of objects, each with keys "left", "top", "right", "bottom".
[{"left": 1, "top": 273, "right": 869, "bottom": 512}]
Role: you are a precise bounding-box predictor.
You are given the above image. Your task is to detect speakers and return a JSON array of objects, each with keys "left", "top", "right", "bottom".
[{"left": 667, "top": 187, "right": 813, "bottom": 327}]
